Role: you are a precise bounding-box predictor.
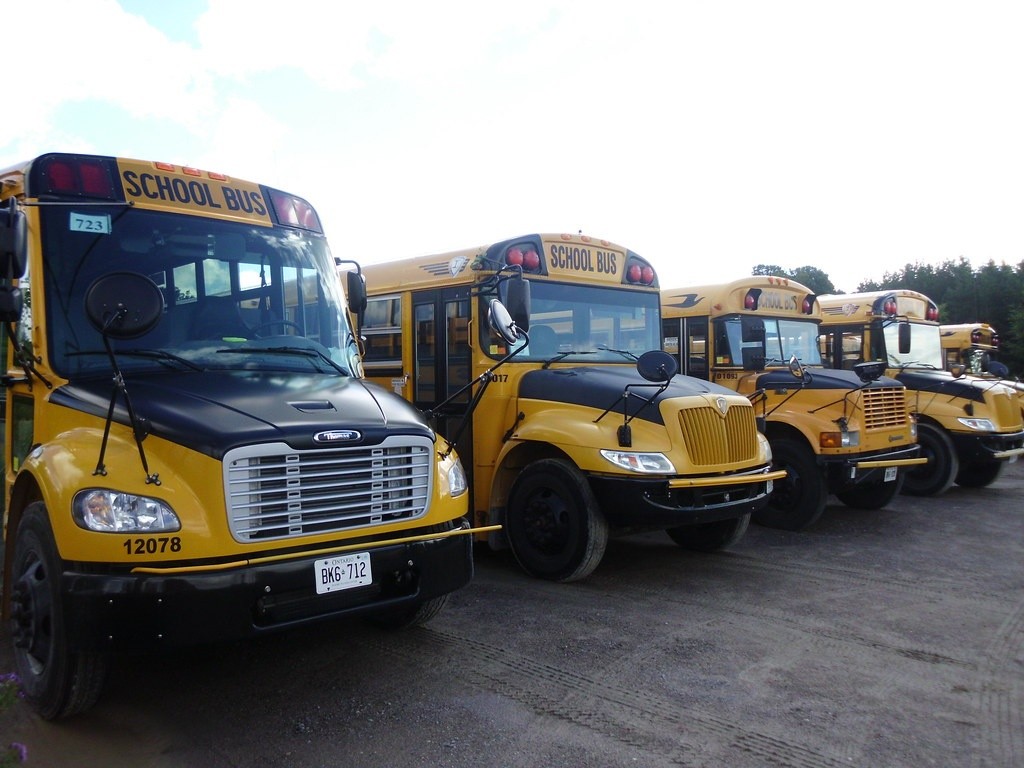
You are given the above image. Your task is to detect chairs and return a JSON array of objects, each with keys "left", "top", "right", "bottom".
[
  {"left": 190, "top": 296, "right": 263, "bottom": 340},
  {"left": 528, "top": 325, "right": 559, "bottom": 357}
]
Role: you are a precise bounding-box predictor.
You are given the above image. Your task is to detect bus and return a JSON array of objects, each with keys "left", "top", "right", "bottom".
[
  {"left": 692, "top": 334, "right": 861, "bottom": 369},
  {"left": 846, "top": 322, "right": 999, "bottom": 372},
  {"left": 659, "top": 273, "right": 929, "bottom": 530},
  {"left": 354, "top": 318, "right": 475, "bottom": 484},
  {"left": 816, "top": 289, "right": 1024, "bottom": 500},
  {"left": 0, "top": 150, "right": 530, "bottom": 727},
  {"left": 279, "top": 234, "right": 789, "bottom": 582}
]
